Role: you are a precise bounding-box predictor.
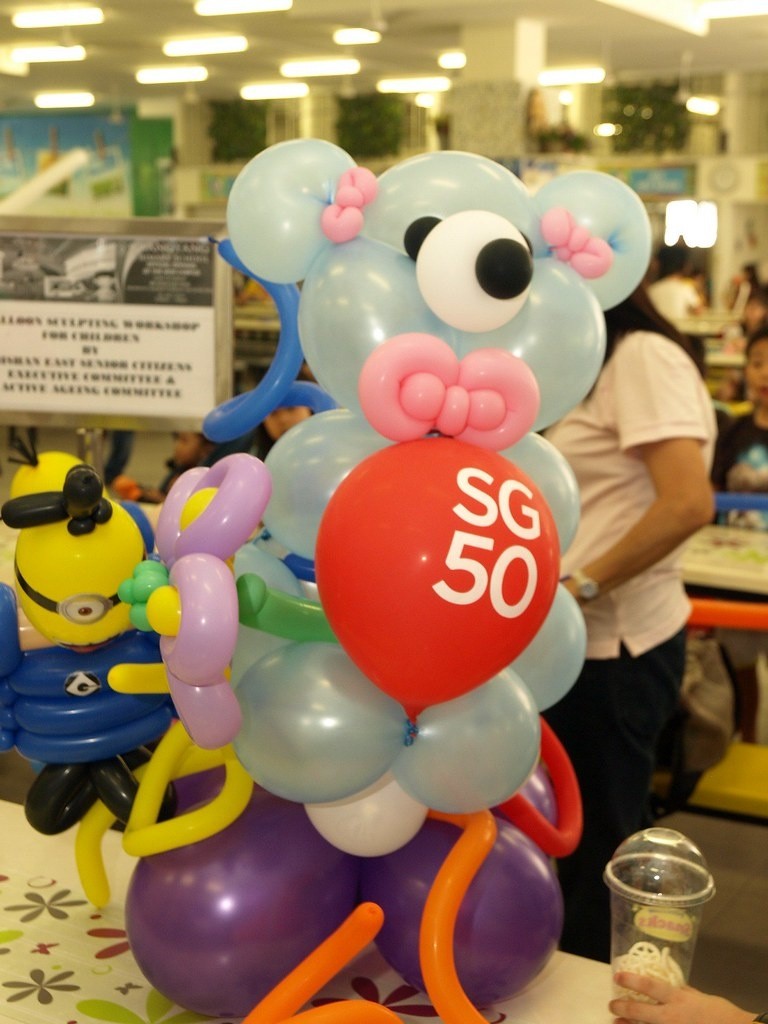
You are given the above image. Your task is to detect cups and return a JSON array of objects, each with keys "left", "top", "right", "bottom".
[{"left": 603, "top": 827, "right": 715, "bottom": 1005}]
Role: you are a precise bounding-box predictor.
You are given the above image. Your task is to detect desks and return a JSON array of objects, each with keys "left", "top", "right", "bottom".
[
  {"left": 0, "top": 796, "right": 663, "bottom": 1024},
  {"left": 673, "top": 523, "right": 766, "bottom": 599},
  {"left": 675, "top": 301, "right": 754, "bottom": 419}
]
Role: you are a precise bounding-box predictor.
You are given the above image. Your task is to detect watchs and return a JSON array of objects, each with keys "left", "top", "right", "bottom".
[{"left": 571, "top": 569, "right": 598, "bottom": 599}]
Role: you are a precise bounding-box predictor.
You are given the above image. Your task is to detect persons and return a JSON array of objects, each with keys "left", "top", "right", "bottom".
[
  {"left": 541, "top": 243, "right": 768, "bottom": 965},
  {"left": 609, "top": 970, "right": 768, "bottom": 1023}
]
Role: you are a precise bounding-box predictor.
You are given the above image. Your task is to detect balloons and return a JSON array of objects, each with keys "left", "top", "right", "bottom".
[{"left": 0, "top": 136, "right": 653, "bottom": 1024}]
